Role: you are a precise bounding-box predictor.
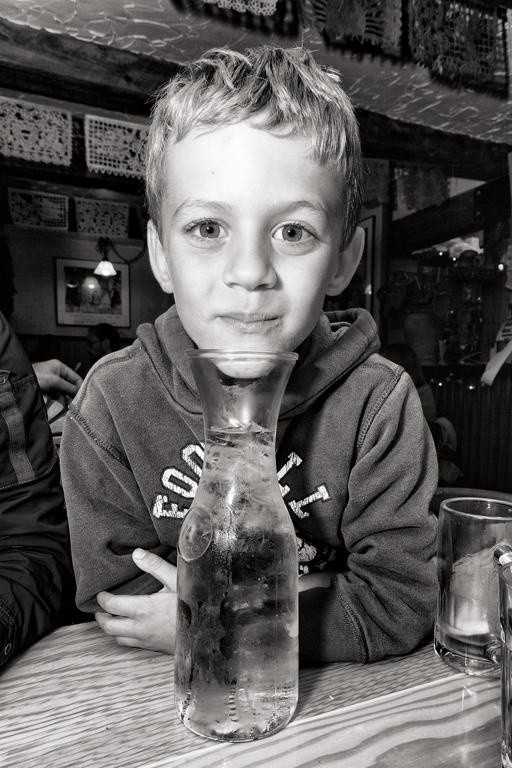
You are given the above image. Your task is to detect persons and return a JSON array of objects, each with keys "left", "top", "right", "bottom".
[
  {"left": 31, "top": 359, "right": 84, "bottom": 398},
  {"left": 57, "top": 44, "right": 438, "bottom": 666},
  {"left": 0, "top": 310, "right": 75, "bottom": 672},
  {"left": 84, "top": 323, "right": 121, "bottom": 359}
]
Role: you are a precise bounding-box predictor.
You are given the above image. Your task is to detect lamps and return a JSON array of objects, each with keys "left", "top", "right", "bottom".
[{"left": 93, "top": 237, "right": 118, "bottom": 278}]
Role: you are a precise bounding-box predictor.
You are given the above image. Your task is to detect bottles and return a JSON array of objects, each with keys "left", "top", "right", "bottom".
[{"left": 171, "top": 349, "right": 302, "bottom": 744}]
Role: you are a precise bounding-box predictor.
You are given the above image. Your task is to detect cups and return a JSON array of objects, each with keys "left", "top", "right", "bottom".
[{"left": 432, "top": 497, "right": 512, "bottom": 768}]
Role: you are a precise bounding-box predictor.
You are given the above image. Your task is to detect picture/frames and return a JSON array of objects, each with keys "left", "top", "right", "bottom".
[{"left": 56, "top": 256, "right": 133, "bottom": 330}]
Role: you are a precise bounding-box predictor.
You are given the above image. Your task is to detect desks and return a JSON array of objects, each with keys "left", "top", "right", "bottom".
[{"left": 0, "top": 618, "right": 512, "bottom": 768}]
